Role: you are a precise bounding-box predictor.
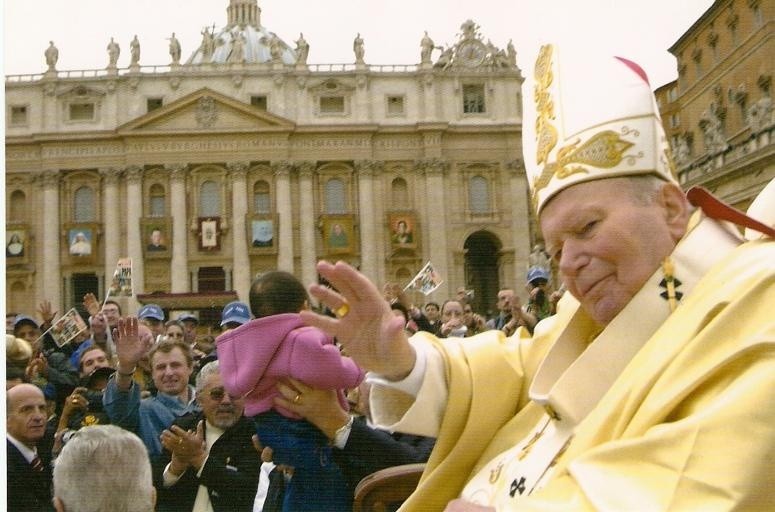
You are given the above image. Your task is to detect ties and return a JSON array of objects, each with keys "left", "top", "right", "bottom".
[{"left": 31, "top": 457, "right": 45, "bottom": 473}]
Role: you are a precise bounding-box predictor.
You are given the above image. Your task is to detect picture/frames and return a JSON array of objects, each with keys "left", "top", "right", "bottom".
[
  {"left": 138, "top": 215, "right": 171, "bottom": 260},
  {"left": 318, "top": 212, "right": 358, "bottom": 255},
  {"left": 6, "top": 221, "right": 30, "bottom": 266},
  {"left": 244, "top": 212, "right": 279, "bottom": 256},
  {"left": 64, "top": 222, "right": 99, "bottom": 261},
  {"left": 383, "top": 209, "right": 422, "bottom": 260},
  {"left": 198, "top": 216, "right": 221, "bottom": 250}
]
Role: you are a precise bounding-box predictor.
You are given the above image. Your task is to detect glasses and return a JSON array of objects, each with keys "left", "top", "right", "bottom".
[{"left": 196, "top": 388, "right": 226, "bottom": 401}]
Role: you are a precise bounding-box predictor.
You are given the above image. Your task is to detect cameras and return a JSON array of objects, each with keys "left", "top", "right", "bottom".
[
  {"left": 77, "top": 390, "right": 105, "bottom": 412},
  {"left": 535, "top": 289, "right": 545, "bottom": 305}
]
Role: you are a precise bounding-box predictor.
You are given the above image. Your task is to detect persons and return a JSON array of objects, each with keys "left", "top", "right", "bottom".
[
  {"left": 203, "top": 229, "right": 216, "bottom": 246},
  {"left": 300, "top": 44, "right": 775, "bottom": 512},
  {"left": 529, "top": 244, "right": 552, "bottom": 273},
  {"left": 106, "top": 38, "right": 119, "bottom": 76},
  {"left": 350, "top": 32, "right": 370, "bottom": 71},
  {"left": 7, "top": 271, "right": 560, "bottom": 512},
  {"left": 70, "top": 233, "right": 91, "bottom": 254},
  {"left": 6, "top": 235, "right": 24, "bottom": 257},
  {"left": 393, "top": 221, "right": 414, "bottom": 243},
  {"left": 330, "top": 225, "right": 347, "bottom": 246},
  {"left": 227, "top": 27, "right": 248, "bottom": 68},
  {"left": 254, "top": 225, "right": 272, "bottom": 248},
  {"left": 419, "top": 26, "right": 434, "bottom": 73},
  {"left": 126, "top": 33, "right": 144, "bottom": 73},
  {"left": 295, "top": 30, "right": 312, "bottom": 71},
  {"left": 42, "top": 39, "right": 60, "bottom": 80},
  {"left": 268, "top": 32, "right": 288, "bottom": 71},
  {"left": 169, "top": 32, "right": 184, "bottom": 72},
  {"left": 198, "top": 21, "right": 217, "bottom": 68},
  {"left": 148, "top": 229, "right": 167, "bottom": 251}
]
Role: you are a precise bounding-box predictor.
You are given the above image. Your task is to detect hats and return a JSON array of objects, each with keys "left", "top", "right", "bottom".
[
  {"left": 220, "top": 301, "right": 251, "bottom": 327},
  {"left": 138, "top": 304, "right": 164, "bottom": 321},
  {"left": 178, "top": 314, "right": 200, "bottom": 326},
  {"left": 13, "top": 315, "right": 38, "bottom": 329},
  {"left": 526, "top": 267, "right": 549, "bottom": 289},
  {"left": 514, "top": 29, "right": 680, "bottom": 220}
]
